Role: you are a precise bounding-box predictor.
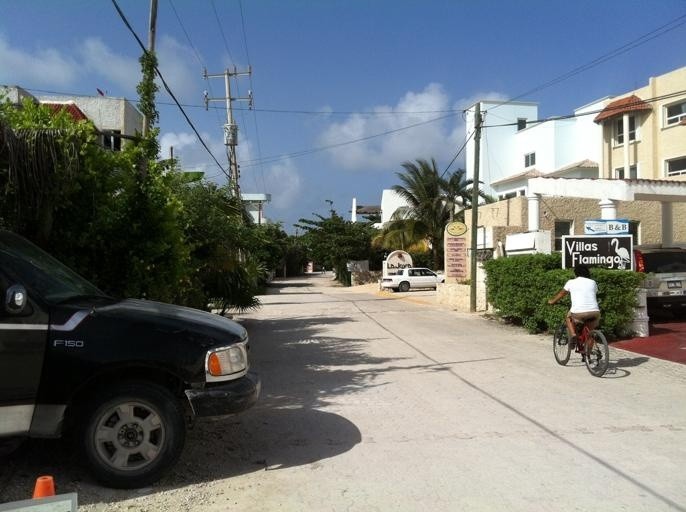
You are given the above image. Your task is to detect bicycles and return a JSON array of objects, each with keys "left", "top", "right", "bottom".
[{"left": 553, "top": 300, "right": 609, "bottom": 377}]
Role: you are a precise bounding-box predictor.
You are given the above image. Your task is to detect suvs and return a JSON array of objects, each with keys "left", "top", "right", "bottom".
[{"left": 633, "top": 243, "right": 685, "bottom": 304}]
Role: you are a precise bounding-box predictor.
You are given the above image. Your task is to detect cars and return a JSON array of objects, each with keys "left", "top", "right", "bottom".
[
  {"left": 382, "top": 268, "right": 444, "bottom": 292},
  {"left": 0, "top": 219, "right": 262, "bottom": 489}
]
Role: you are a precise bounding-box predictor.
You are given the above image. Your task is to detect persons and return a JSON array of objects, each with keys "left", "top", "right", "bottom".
[{"left": 547, "top": 262, "right": 603, "bottom": 365}]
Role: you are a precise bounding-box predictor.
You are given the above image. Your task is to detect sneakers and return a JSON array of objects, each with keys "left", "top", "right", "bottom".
[{"left": 568, "top": 336, "right": 577, "bottom": 350}]
